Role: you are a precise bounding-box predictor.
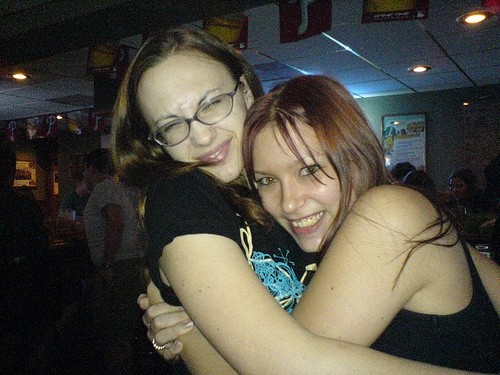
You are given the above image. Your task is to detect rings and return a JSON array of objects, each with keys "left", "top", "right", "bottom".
[{"left": 152, "top": 339, "right": 166, "bottom": 349}]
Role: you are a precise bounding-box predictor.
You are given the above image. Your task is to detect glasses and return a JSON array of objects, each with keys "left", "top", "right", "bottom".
[{"left": 147, "top": 80, "right": 242, "bottom": 148}]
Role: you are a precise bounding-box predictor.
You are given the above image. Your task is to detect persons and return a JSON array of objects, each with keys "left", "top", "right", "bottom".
[
  {"left": 392, "top": 163, "right": 416, "bottom": 186},
  {"left": 446, "top": 167, "right": 483, "bottom": 218},
  {"left": 480, "top": 154, "right": 500, "bottom": 267},
  {"left": 58, "top": 174, "right": 90, "bottom": 217},
  {"left": 0, "top": 142, "right": 75, "bottom": 375},
  {"left": 108, "top": 24, "right": 500, "bottom": 375},
  {"left": 402, "top": 170, "right": 437, "bottom": 202},
  {"left": 82, "top": 148, "right": 144, "bottom": 375}
]
